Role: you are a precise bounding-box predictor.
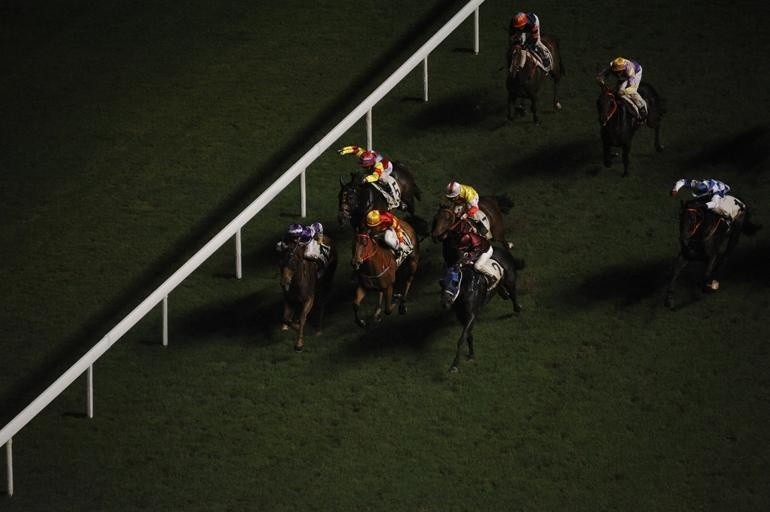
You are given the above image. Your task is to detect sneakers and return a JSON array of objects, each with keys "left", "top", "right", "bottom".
[
  {"left": 484, "top": 273, "right": 496, "bottom": 288},
  {"left": 543, "top": 53, "right": 551, "bottom": 67},
  {"left": 640, "top": 102, "right": 648, "bottom": 115},
  {"left": 723, "top": 216, "right": 732, "bottom": 233}
]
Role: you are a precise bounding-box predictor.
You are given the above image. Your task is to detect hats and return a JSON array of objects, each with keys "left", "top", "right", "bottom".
[
  {"left": 358, "top": 152, "right": 375, "bottom": 168},
  {"left": 445, "top": 181, "right": 461, "bottom": 198},
  {"left": 366, "top": 208, "right": 384, "bottom": 227},
  {"left": 455, "top": 233, "right": 481, "bottom": 249},
  {"left": 510, "top": 12, "right": 529, "bottom": 28},
  {"left": 288, "top": 223, "right": 303, "bottom": 234},
  {"left": 690, "top": 182, "right": 709, "bottom": 198},
  {"left": 612, "top": 56, "right": 627, "bottom": 72}
]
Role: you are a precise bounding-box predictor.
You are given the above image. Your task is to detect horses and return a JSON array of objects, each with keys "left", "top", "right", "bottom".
[
  {"left": 429, "top": 190, "right": 515, "bottom": 243},
  {"left": 505, "top": 32, "right": 567, "bottom": 126},
  {"left": 279, "top": 233, "right": 339, "bottom": 352},
  {"left": 596, "top": 81, "right": 668, "bottom": 179},
  {"left": 350, "top": 215, "right": 429, "bottom": 327},
  {"left": 337, "top": 167, "right": 422, "bottom": 232},
  {"left": 664, "top": 198, "right": 762, "bottom": 308},
  {"left": 438, "top": 244, "right": 526, "bottom": 375}
]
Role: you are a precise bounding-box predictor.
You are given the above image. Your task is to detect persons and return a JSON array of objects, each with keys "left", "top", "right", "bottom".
[
  {"left": 444, "top": 179, "right": 494, "bottom": 239},
  {"left": 504, "top": 11, "right": 554, "bottom": 75},
  {"left": 595, "top": 55, "right": 650, "bottom": 128},
  {"left": 275, "top": 220, "right": 333, "bottom": 282},
  {"left": 454, "top": 231, "right": 505, "bottom": 294},
  {"left": 337, "top": 143, "right": 409, "bottom": 212},
  {"left": 364, "top": 209, "right": 416, "bottom": 266},
  {"left": 669, "top": 176, "right": 738, "bottom": 233}
]
